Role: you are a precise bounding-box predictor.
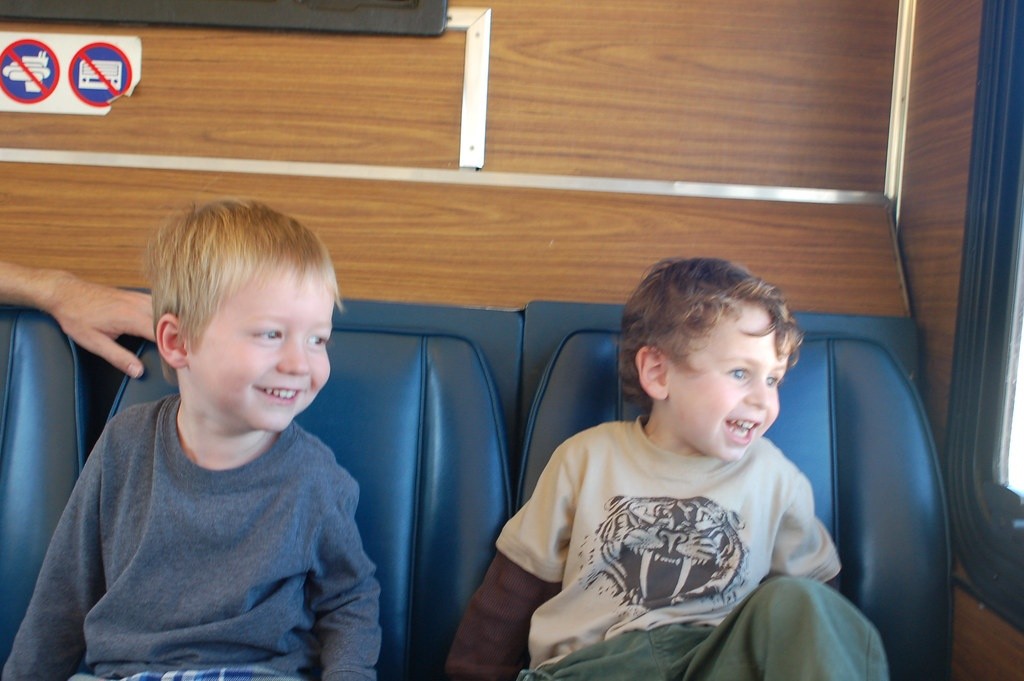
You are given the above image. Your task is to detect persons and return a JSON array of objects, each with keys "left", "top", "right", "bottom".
[
  {"left": 0, "top": 261, "right": 158, "bottom": 378},
  {"left": 0, "top": 197, "right": 381, "bottom": 681},
  {"left": 443, "top": 256, "right": 889, "bottom": 681}
]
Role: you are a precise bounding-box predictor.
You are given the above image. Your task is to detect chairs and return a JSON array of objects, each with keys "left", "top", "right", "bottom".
[
  {"left": 104, "top": 327, "right": 510, "bottom": 681},
  {"left": 0, "top": 303, "right": 87, "bottom": 670},
  {"left": 517, "top": 329, "right": 954, "bottom": 681}
]
